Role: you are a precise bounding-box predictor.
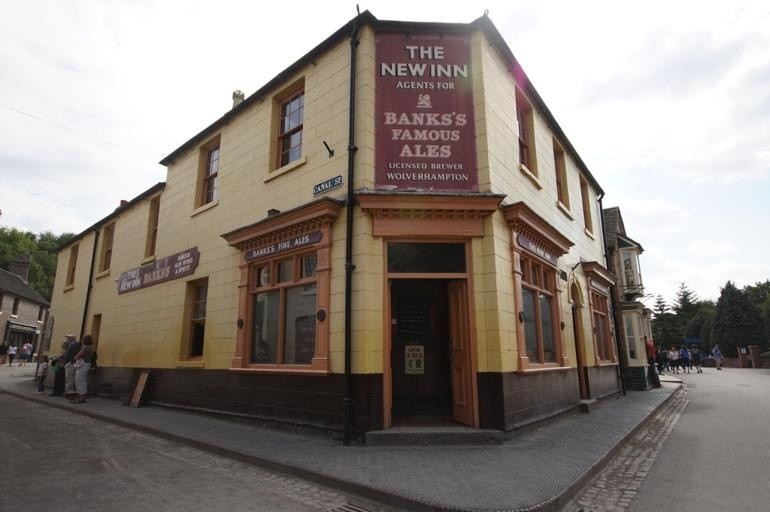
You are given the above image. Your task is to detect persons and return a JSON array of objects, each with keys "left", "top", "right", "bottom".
[
  {"left": 18, "top": 339, "right": 31, "bottom": 366},
  {"left": 48, "top": 340, "right": 69, "bottom": 395},
  {"left": 35, "top": 355, "right": 49, "bottom": 394},
  {"left": 73, "top": 334, "right": 94, "bottom": 403},
  {"left": 0, "top": 341, "right": 7, "bottom": 364},
  {"left": 64, "top": 332, "right": 80, "bottom": 397},
  {"left": 6, "top": 340, "right": 19, "bottom": 367},
  {"left": 655, "top": 344, "right": 726, "bottom": 376}
]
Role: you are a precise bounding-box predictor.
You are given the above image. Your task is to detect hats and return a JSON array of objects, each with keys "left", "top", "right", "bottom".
[{"left": 64, "top": 333, "right": 78, "bottom": 339}]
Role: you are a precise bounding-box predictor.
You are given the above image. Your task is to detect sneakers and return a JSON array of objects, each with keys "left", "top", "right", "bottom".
[{"left": 32, "top": 391, "right": 86, "bottom": 403}]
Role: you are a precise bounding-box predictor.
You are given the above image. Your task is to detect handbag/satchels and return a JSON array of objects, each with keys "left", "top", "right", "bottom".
[{"left": 90, "top": 351, "right": 98, "bottom": 366}]
